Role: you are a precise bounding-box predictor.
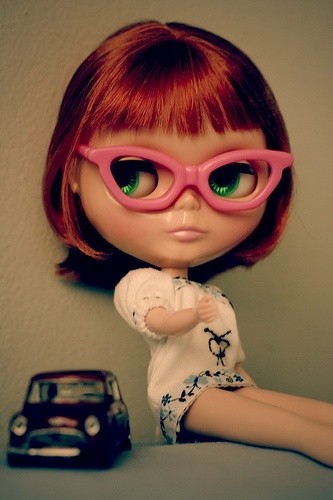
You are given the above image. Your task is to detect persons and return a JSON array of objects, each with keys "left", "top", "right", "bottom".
[{"left": 42, "top": 20, "right": 333, "bottom": 468}]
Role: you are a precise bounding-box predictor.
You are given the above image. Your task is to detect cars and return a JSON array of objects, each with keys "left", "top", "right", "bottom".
[{"left": 6, "top": 368, "right": 131, "bottom": 470}]
